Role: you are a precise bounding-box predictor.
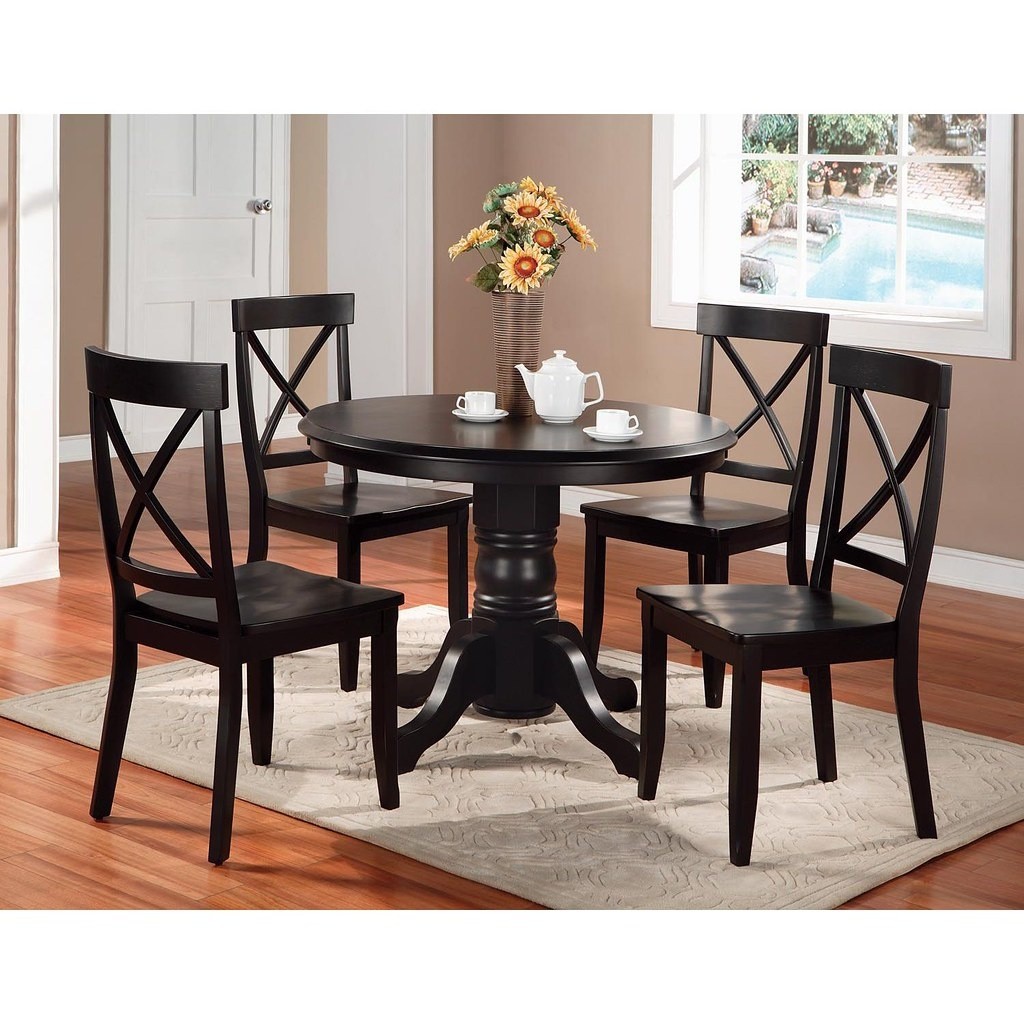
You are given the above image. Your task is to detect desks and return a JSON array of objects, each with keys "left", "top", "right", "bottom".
[{"left": 299, "top": 393, "right": 737, "bottom": 781}]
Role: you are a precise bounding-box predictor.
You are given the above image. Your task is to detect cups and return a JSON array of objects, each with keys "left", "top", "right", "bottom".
[
  {"left": 457, "top": 391, "right": 496, "bottom": 414},
  {"left": 596, "top": 409, "right": 639, "bottom": 435}
]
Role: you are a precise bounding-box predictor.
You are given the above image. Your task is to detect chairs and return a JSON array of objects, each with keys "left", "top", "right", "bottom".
[
  {"left": 580, "top": 303, "right": 831, "bottom": 667},
  {"left": 635, "top": 344, "right": 952, "bottom": 869},
  {"left": 232, "top": 292, "right": 473, "bottom": 693},
  {"left": 83, "top": 344, "right": 405, "bottom": 862}
]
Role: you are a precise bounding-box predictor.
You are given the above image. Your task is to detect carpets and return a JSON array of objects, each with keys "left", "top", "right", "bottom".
[{"left": 0, "top": 602, "right": 1024, "bottom": 910}]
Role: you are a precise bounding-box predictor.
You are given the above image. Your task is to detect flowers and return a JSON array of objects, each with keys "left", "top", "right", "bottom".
[
  {"left": 809, "top": 160, "right": 831, "bottom": 182},
  {"left": 448, "top": 176, "right": 598, "bottom": 295},
  {"left": 744, "top": 198, "right": 774, "bottom": 219},
  {"left": 852, "top": 162, "right": 886, "bottom": 184},
  {"left": 831, "top": 162, "right": 847, "bottom": 182}
]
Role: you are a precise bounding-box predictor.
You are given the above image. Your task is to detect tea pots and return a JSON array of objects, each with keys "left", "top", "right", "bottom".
[{"left": 513, "top": 350, "right": 604, "bottom": 423}]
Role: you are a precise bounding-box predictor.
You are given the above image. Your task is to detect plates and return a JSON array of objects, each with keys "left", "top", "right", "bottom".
[
  {"left": 451, "top": 408, "right": 508, "bottom": 422},
  {"left": 583, "top": 426, "right": 643, "bottom": 443}
]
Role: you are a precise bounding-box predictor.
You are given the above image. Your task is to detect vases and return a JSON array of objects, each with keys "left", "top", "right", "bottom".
[
  {"left": 857, "top": 181, "right": 875, "bottom": 198},
  {"left": 829, "top": 180, "right": 847, "bottom": 197},
  {"left": 491, "top": 290, "right": 544, "bottom": 420},
  {"left": 752, "top": 215, "right": 772, "bottom": 235},
  {"left": 808, "top": 181, "right": 824, "bottom": 199}
]
